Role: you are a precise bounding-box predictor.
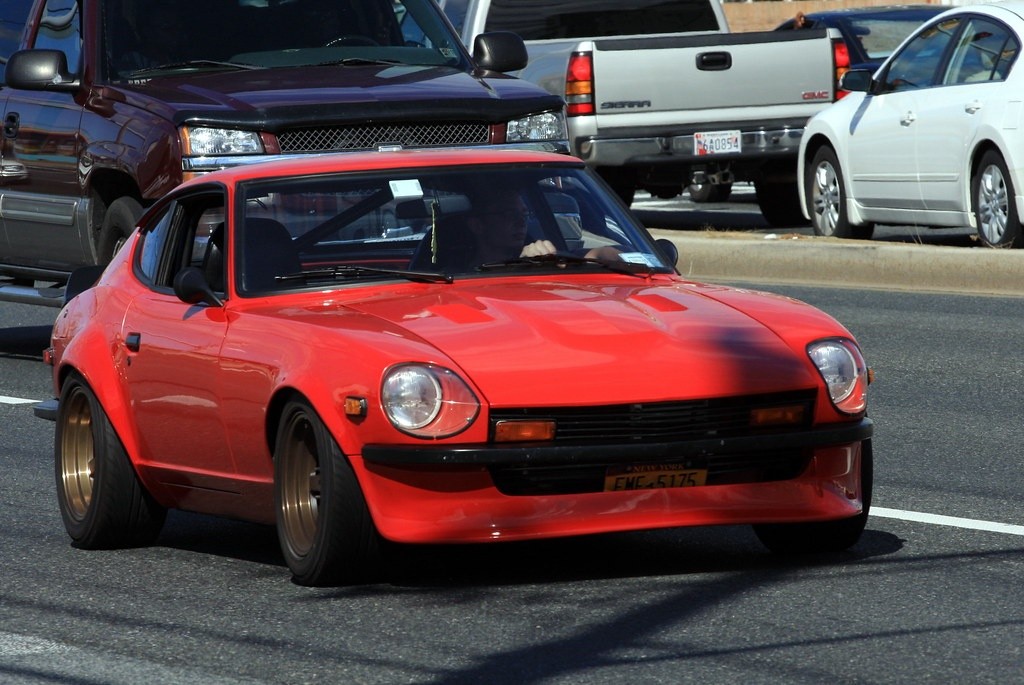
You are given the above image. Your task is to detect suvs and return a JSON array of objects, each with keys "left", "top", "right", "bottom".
[{"left": 1, "top": 0, "right": 572, "bottom": 306}]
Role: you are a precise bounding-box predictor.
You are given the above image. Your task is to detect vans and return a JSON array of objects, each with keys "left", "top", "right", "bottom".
[
  {"left": 796, "top": 6, "right": 1024, "bottom": 246},
  {"left": 769, "top": 0, "right": 1024, "bottom": 92}
]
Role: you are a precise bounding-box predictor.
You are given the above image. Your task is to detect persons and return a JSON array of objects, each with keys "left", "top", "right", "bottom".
[{"left": 454, "top": 185, "right": 630, "bottom": 272}]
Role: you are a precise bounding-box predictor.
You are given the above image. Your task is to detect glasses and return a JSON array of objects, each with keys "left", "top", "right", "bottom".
[{"left": 475, "top": 210, "right": 533, "bottom": 225}]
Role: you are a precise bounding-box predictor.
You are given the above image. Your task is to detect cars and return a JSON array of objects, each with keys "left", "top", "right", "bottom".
[{"left": 42, "top": 143, "right": 878, "bottom": 582}]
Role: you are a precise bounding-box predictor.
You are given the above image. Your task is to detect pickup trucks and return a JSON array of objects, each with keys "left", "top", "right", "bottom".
[{"left": 457, "top": 0, "right": 843, "bottom": 228}]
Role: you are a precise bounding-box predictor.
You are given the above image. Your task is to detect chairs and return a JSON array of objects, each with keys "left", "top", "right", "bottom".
[
  {"left": 407, "top": 214, "right": 475, "bottom": 273},
  {"left": 201, "top": 217, "right": 308, "bottom": 292}
]
